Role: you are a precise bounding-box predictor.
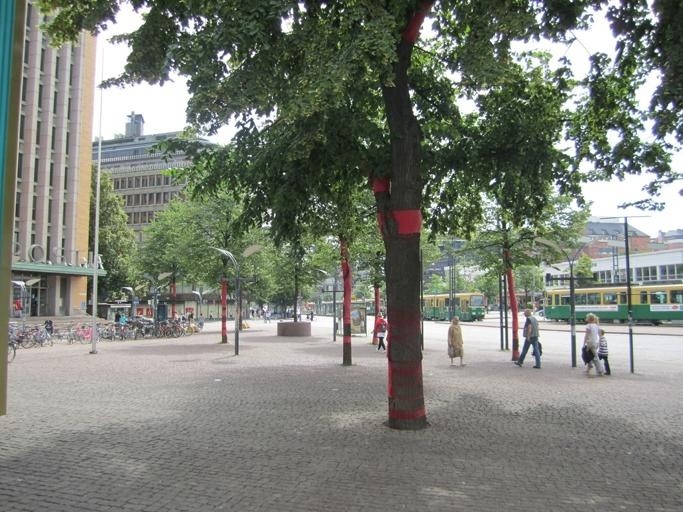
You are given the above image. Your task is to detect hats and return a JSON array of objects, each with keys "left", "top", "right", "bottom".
[{"left": 376, "top": 311, "right": 383, "bottom": 317}]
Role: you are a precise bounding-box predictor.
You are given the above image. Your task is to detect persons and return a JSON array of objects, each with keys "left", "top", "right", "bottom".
[
  {"left": 375, "top": 312, "right": 388, "bottom": 353},
  {"left": 581, "top": 313, "right": 604, "bottom": 376},
  {"left": 512, "top": 309, "right": 541, "bottom": 369},
  {"left": 113, "top": 303, "right": 270, "bottom": 333},
  {"left": 447, "top": 316, "right": 466, "bottom": 367},
  {"left": 596, "top": 328, "right": 611, "bottom": 375}
]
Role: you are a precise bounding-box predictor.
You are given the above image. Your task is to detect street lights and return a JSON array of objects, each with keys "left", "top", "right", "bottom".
[{"left": 559, "top": 237, "right": 609, "bottom": 368}]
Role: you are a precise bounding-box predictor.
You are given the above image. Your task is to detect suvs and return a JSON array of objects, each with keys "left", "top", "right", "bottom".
[{"left": 525, "top": 298, "right": 552, "bottom": 310}]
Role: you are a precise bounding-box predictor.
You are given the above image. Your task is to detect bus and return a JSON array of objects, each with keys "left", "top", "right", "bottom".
[
  {"left": 419, "top": 292, "right": 485, "bottom": 323},
  {"left": 84, "top": 302, "right": 132, "bottom": 321},
  {"left": 539, "top": 283, "right": 683, "bottom": 328},
  {"left": 353, "top": 298, "right": 386, "bottom": 318}
]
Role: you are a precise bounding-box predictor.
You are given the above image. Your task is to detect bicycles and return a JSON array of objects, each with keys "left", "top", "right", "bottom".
[{"left": 7, "top": 314, "right": 199, "bottom": 362}]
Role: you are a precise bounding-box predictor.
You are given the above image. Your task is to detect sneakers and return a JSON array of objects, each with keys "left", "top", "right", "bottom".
[
  {"left": 596, "top": 372, "right": 609, "bottom": 376},
  {"left": 533, "top": 365, "right": 540, "bottom": 369},
  {"left": 514, "top": 361, "right": 522, "bottom": 366},
  {"left": 375, "top": 348, "right": 387, "bottom": 353},
  {"left": 583, "top": 369, "right": 589, "bottom": 374},
  {"left": 449, "top": 363, "right": 467, "bottom": 368}
]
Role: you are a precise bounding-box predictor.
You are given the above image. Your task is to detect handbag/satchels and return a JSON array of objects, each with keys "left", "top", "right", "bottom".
[
  {"left": 531, "top": 342, "right": 543, "bottom": 356},
  {"left": 581, "top": 346, "right": 595, "bottom": 365}
]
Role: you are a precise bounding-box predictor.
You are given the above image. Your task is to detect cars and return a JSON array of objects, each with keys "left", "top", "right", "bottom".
[{"left": 538, "top": 310, "right": 543, "bottom": 317}]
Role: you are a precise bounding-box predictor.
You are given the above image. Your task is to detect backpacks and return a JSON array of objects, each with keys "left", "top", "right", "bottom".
[{"left": 380, "top": 323, "right": 387, "bottom": 332}]
